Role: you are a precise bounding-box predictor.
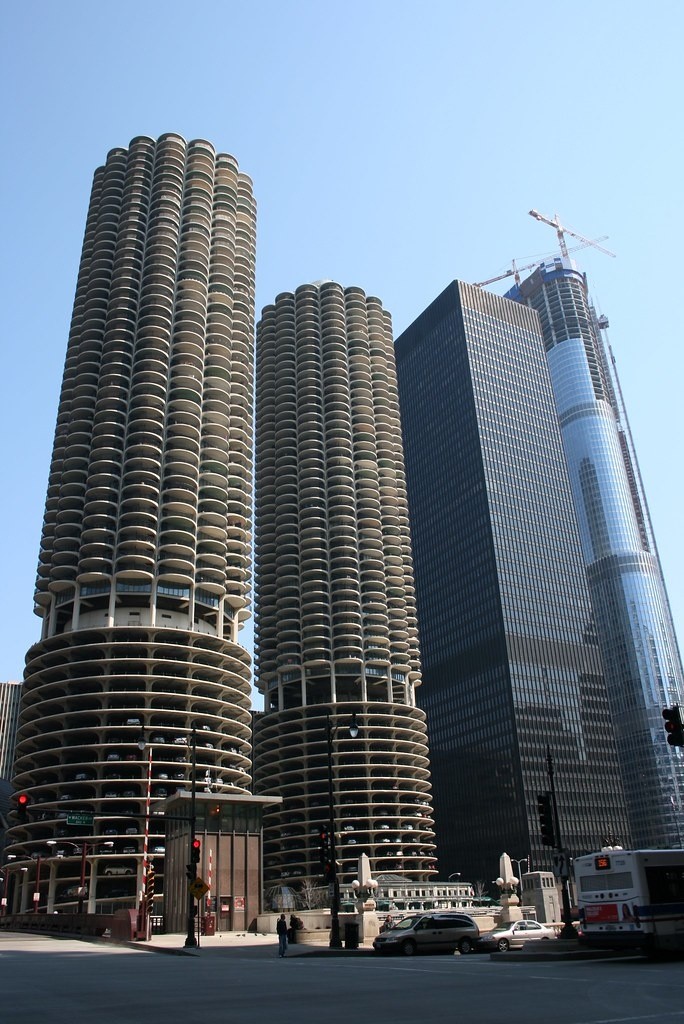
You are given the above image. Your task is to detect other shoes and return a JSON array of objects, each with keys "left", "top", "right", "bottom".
[
  {"left": 279, "top": 955, "right": 282, "bottom": 958},
  {"left": 283, "top": 954, "right": 286, "bottom": 957}
]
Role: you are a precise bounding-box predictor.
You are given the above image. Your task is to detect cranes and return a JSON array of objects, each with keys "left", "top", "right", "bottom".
[
  {"left": 526, "top": 206, "right": 620, "bottom": 261},
  {"left": 469, "top": 232, "right": 611, "bottom": 287}
]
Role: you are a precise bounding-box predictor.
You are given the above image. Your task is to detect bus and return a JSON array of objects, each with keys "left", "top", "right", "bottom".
[{"left": 572, "top": 845, "right": 684, "bottom": 958}]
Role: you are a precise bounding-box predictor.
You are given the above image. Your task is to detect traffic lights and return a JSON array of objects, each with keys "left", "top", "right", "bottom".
[
  {"left": 16, "top": 795, "right": 27, "bottom": 821},
  {"left": 184, "top": 862, "right": 197, "bottom": 881},
  {"left": 323, "top": 860, "right": 333, "bottom": 883},
  {"left": 317, "top": 830, "right": 329, "bottom": 868},
  {"left": 537, "top": 791, "right": 556, "bottom": 846},
  {"left": 661, "top": 704, "right": 684, "bottom": 748},
  {"left": 191, "top": 838, "right": 201, "bottom": 864}
]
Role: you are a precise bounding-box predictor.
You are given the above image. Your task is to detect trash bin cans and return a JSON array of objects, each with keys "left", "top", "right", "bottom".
[{"left": 344, "top": 921, "right": 361, "bottom": 949}]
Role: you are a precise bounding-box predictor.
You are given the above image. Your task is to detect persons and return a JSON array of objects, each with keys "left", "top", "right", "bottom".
[
  {"left": 287, "top": 914, "right": 299, "bottom": 944},
  {"left": 384, "top": 915, "right": 395, "bottom": 932},
  {"left": 276, "top": 914, "right": 289, "bottom": 958}
]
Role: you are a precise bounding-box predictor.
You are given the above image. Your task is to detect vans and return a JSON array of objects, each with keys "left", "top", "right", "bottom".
[{"left": 371, "top": 912, "right": 480, "bottom": 956}]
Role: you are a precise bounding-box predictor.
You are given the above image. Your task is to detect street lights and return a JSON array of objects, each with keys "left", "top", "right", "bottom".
[
  {"left": 46, "top": 841, "right": 115, "bottom": 914},
  {"left": 0, "top": 867, "right": 28, "bottom": 916},
  {"left": 6, "top": 855, "right": 64, "bottom": 914},
  {"left": 446, "top": 872, "right": 462, "bottom": 883},
  {"left": 510, "top": 857, "right": 528, "bottom": 904},
  {"left": 135, "top": 719, "right": 200, "bottom": 948},
  {"left": 324, "top": 709, "right": 362, "bottom": 949}
]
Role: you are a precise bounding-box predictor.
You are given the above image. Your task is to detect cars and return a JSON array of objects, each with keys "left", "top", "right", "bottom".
[
  {"left": 99, "top": 847, "right": 113, "bottom": 854},
  {"left": 475, "top": 918, "right": 557, "bottom": 951},
  {"left": 264, "top": 799, "right": 436, "bottom": 881},
  {"left": 103, "top": 886, "right": 134, "bottom": 898},
  {"left": 31, "top": 851, "right": 47, "bottom": 859},
  {"left": 36, "top": 718, "right": 246, "bottom": 837},
  {"left": 122, "top": 847, "right": 136, "bottom": 853},
  {"left": 57, "top": 850, "right": 66, "bottom": 857},
  {"left": 102, "top": 863, "right": 135, "bottom": 875},
  {"left": 150, "top": 846, "right": 167, "bottom": 854},
  {"left": 72, "top": 846, "right": 86, "bottom": 857}
]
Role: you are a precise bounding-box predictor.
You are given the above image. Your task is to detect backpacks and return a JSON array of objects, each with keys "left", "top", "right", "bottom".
[{"left": 295, "top": 917, "right": 304, "bottom": 929}]
